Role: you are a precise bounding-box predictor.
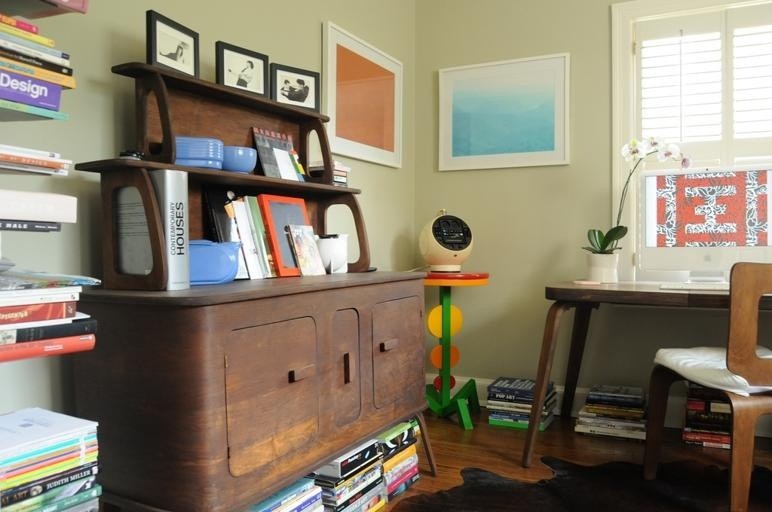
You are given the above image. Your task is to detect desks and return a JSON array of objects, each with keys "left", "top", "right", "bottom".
[
  {"left": 519, "top": 280, "right": 772, "bottom": 468},
  {"left": 422, "top": 272, "right": 490, "bottom": 432}
]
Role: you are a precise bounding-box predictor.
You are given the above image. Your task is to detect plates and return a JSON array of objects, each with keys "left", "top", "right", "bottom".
[{"left": 174, "top": 135, "right": 224, "bottom": 169}]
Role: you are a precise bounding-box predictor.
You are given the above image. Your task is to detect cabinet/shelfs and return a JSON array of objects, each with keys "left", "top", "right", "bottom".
[
  {"left": 66, "top": 61, "right": 437, "bottom": 512},
  {"left": 0, "top": 0, "right": 101, "bottom": 512}
]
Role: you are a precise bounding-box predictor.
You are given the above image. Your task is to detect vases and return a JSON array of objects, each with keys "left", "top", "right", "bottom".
[{"left": 586, "top": 253, "right": 619, "bottom": 284}]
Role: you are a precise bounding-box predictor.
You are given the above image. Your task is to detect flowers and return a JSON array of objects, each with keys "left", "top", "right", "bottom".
[{"left": 582, "top": 136, "right": 692, "bottom": 255}]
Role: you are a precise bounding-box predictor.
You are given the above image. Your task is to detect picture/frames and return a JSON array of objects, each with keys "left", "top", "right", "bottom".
[
  {"left": 215, "top": 40, "right": 269, "bottom": 100},
  {"left": 270, "top": 61, "right": 321, "bottom": 113},
  {"left": 438, "top": 52, "right": 570, "bottom": 172},
  {"left": 146, "top": 9, "right": 200, "bottom": 80},
  {"left": 323, "top": 19, "right": 403, "bottom": 171}
]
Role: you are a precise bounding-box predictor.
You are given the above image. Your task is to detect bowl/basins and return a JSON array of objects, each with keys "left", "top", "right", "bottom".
[
  {"left": 223, "top": 145, "right": 257, "bottom": 174},
  {"left": 188, "top": 242, "right": 242, "bottom": 286}
]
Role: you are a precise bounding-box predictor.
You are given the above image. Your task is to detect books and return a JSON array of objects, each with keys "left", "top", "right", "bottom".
[
  {"left": 0, "top": 188, "right": 77, "bottom": 233},
  {"left": 485, "top": 375, "right": 559, "bottom": 434},
  {"left": 682, "top": 391, "right": 736, "bottom": 450},
  {"left": 573, "top": 382, "right": 645, "bottom": 441},
  {"left": 113, "top": 170, "right": 192, "bottom": 292},
  {"left": 210, "top": 189, "right": 328, "bottom": 281},
  {"left": 252, "top": 416, "right": 421, "bottom": 512},
  {"left": 0, "top": 405, "right": 103, "bottom": 512},
  {"left": 0, "top": 13, "right": 78, "bottom": 123},
  {"left": 0, "top": 257, "right": 104, "bottom": 362},
  {"left": 310, "top": 162, "right": 351, "bottom": 191},
  {"left": 0, "top": 139, "right": 74, "bottom": 178},
  {"left": 251, "top": 123, "right": 306, "bottom": 183}
]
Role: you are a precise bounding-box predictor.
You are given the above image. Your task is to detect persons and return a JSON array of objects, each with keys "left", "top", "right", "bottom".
[
  {"left": 229, "top": 60, "right": 255, "bottom": 88},
  {"left": 161, "top": 41, "right": 188, "bottom": 63},
  {"left": 281, "top": 76, "right": 310, "bottom": 105}
]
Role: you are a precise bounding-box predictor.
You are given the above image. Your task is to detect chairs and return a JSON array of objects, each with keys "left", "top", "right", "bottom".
[{"left": 643, "top": 261, "right": 772, "bottom": 512}]
